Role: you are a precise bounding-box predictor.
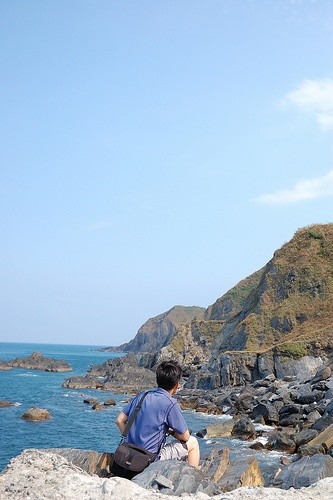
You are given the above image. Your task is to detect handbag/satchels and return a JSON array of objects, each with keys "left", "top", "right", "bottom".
[{"left": 108, "top": 442, "right": 156, "bottom": 480}]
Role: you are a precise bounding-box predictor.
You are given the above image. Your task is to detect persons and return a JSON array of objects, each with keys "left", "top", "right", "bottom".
[{"left": 114, "top": 360, "right": 201, "bottom": 468}]
